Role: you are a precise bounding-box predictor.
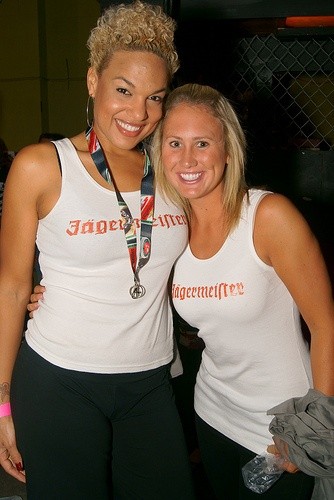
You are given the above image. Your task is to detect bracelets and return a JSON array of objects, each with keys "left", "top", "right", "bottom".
[{"left": 0, "top": 402, "right": 11, "bottom": 418}]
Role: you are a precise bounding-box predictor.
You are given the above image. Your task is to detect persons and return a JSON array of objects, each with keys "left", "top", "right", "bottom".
[
  {"left": 26, "top": 83, "right": 334, "bottom": 500},
  {"left": 0, "top": 138, "right": 15, "bottom": 221},
  {"left": 0, "top": 0, "right": 193, "bottom": 500},
  {"left": 37, "top": 131, "right": 64, "bottom": 144}
]
